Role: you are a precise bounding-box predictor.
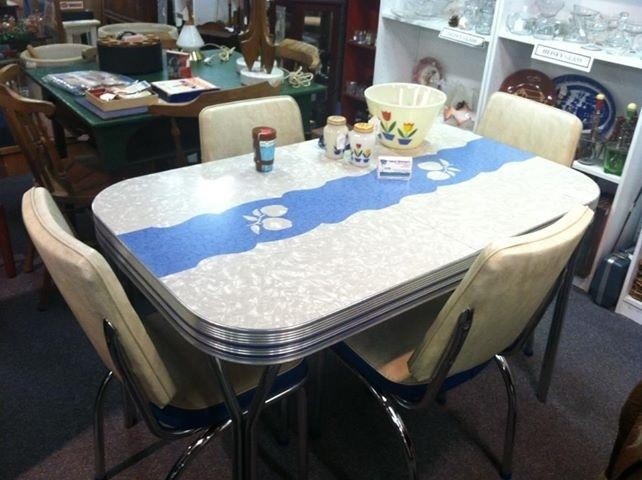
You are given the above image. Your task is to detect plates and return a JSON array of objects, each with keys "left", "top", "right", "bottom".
[
  {"left": 409, "top": 55, "right": 444, "bottom": 89},
  {"left": 552, "top": 74, "right": 618, "bottom": 136},
  {"left": 498, "top": 69, "right": 555, "bottom": 107},
  {"left": 235, "top": 57, "right": 278, "bottom": 74},
  {"left": 238, "top": 68, "right": 284, "bottom": 88}
]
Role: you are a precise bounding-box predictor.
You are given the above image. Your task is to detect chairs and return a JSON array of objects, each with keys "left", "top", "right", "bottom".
[{"left": 0, "top": 63, "right": 128, "bottom": 273}]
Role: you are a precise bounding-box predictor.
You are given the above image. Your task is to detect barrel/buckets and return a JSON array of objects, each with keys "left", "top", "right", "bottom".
[
  {"left": 21, "top": 42, "right": 95, "bottom": 140},
  {"left": 97, "top": 22, "right": 178, "bottom": 50}
]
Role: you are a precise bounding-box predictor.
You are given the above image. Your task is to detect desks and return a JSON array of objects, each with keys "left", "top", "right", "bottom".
[
  {"left": 23, "top": 48, "right": 328, "bottom": 171},
  {"left": 93, "top": 126, "right": 603, "bottom": 476}
]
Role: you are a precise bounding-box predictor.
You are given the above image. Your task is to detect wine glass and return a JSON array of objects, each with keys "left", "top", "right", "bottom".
[{"left": 505, "top": 0, "right": 642, "bottom": 59}]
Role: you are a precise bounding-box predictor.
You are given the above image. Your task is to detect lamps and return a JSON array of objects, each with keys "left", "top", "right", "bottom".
[{"left": 174, "top": 23, "right": 204, "bottom": 62}]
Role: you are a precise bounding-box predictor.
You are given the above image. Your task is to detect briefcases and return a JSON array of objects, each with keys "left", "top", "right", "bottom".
[{"left": 590, "top": 247, "right": 636, "bottom": 309}]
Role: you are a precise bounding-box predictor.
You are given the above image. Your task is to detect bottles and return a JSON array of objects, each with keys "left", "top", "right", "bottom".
[
  {"left": 577, "top": 94, "right": 637, "bottom": 176},
  {"left": 321, "top": 114, "right": 374, "bottom": 168}
]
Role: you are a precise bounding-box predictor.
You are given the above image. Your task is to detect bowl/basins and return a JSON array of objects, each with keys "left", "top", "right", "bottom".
[
  {"left": 18, "top": 43, "right": 92, "bottom": 100},
  {"left": 364, "top": 82, "right": 447, "bottom": 150}
]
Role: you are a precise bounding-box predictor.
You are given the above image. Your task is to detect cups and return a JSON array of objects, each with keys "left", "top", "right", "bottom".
[
  {"left": 252, "top": 127, "right": 277, "bottom": 173},
  {"left": 392, "top": 0, "right": 493, "bottom": 38}
]
